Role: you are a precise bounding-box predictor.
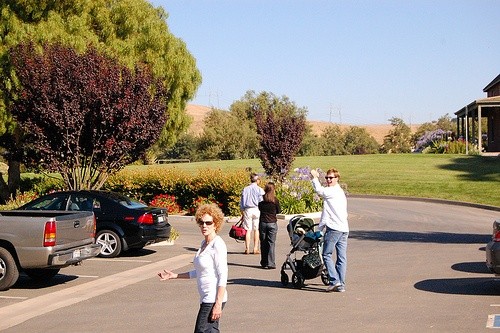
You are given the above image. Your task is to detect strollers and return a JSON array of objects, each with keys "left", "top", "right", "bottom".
[{"left": 280, "top": 214, "right": 331, "bottom": 288}]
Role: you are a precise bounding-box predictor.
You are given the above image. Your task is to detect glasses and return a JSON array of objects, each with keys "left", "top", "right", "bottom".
[
  {"left": 325, "top": 176, "right": 337, "bottom": 180},
  {"left": 199, "top": 221, "right": 214, "bottom": 225}
]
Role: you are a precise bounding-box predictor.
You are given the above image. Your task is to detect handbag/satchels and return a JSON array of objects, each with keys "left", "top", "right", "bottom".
[{"left": 229, "top": 219, "right": 247, "bottom": 240}]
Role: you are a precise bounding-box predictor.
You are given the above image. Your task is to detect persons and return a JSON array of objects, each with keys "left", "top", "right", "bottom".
[
  {"left": 158, "top": 205, "right": 228, "bottom": 333},
  {"left": 310, "top": 168, "right": 348, "bottom": 291},
  {"left": 258, "top": 182, "right": 281, "bottom": 268},
  {"left": 240, "top": 173, "right": 265, "bottom": 254}
]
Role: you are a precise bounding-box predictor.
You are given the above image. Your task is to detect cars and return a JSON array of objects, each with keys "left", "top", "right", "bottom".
[{"left": 11, "top": 189, "right": 172, "bottom": 259}]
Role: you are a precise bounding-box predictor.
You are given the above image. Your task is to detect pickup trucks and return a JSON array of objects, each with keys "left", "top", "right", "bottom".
[{"left": 0, "top": 210, "right": 102, "bottom": 292}]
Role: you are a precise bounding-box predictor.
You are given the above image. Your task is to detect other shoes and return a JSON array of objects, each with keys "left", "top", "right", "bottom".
[
  {"left": 326, "top": 283, "right": 341, "bottom": 292},
  {"left": 332, "top": 286, "right": 345, "bottom": 292},
  {"left": 260, "top": 261, "right": 268, "bottom": 268},
  {"left": 253, "top": 250, "right": 260, "bottom": 254},
  {"left": 245, "top": 249, "right": 250, "bottom": 254},
  {"left": 268, "top": 265, "right": 276, "bottom": 269}
]
